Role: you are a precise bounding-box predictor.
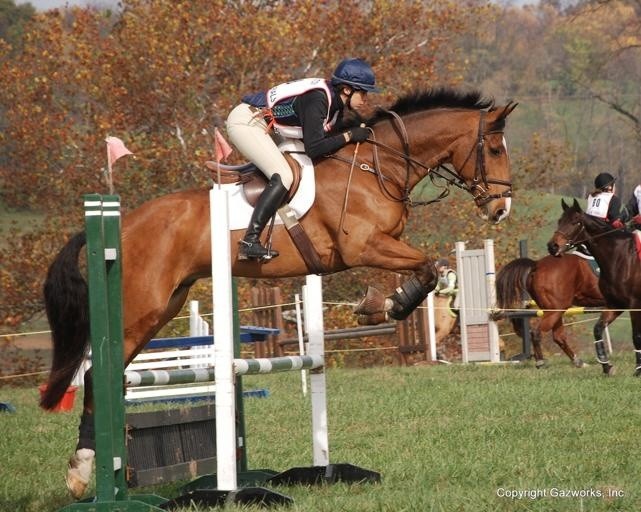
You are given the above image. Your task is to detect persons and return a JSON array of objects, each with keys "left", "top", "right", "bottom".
[
  {"left": 612, "top": 184, "right": 641, "bottom": 261},
  {"left": 434, "top": 258, "right": 459, "bottom": 316},
  {"left": 226, "top": 59, "right": 384, "bottom": 262},
  {"left": 585, "top": 173, "right": 621, "bottom": 259}
]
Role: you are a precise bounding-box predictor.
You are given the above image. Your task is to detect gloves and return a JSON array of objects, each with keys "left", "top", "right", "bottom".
[
  {"left": 632, "top": 215, "right": 641, "bottom": 224},
  {"left": 347, "top": 124, "right": 369, "bottom": 142},
  {"left": 612, "top": 220, "right": 624, "bottom": 229}
]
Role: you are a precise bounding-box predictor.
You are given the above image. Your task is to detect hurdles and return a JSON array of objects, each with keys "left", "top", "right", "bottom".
[
  {"left": 247, "top": 238, "right": 611, "bottom": 371},
  {"left": 63, "top": 190, "right": 381, "bottom": 512}
]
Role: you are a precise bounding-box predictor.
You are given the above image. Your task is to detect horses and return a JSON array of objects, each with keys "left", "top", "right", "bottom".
[
  {"left": 547, "top": 196, "right": 641, "bottom": 378},
  {"left": 38, "top": 81, "right": 521, "bottom": 501},
  {"left": 433, "top": 290, "right": 507, "bottom": 353},
  {"left": 495, "top": 255, "right": 602, "bottom": 369}
]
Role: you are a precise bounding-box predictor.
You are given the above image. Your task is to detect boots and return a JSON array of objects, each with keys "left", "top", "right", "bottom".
[{"left": 238, "top": 174, "right": 287, "bottom": 260}]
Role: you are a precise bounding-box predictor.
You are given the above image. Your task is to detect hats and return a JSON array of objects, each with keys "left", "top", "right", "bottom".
[{"left": 596, "top": 173, "right": 617, "bottom": 192}]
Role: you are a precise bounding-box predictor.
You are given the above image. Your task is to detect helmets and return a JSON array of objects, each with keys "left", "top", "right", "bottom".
[
  {"left": 333, "top": 58, "right": 380, "bottom": 94},
  {"left": 436, "top": 259, "right": 450, "bottom": 267}
]
